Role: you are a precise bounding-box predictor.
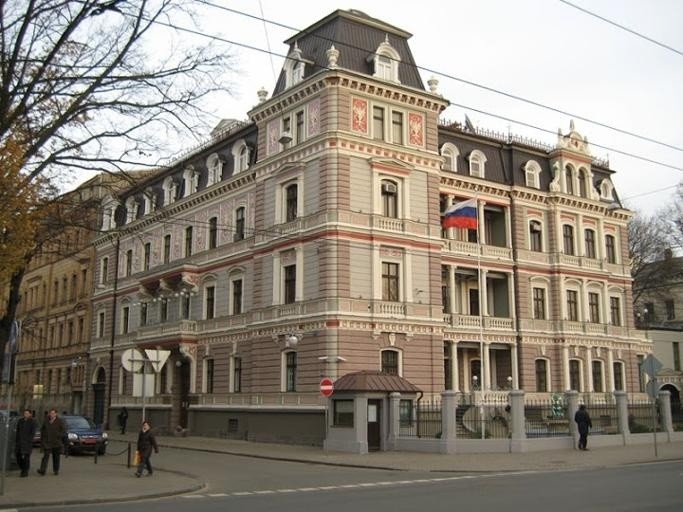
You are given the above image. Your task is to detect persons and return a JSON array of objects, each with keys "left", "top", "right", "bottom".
[
  {"left": 14, "top": 409, "right": 36, "bottom": 477},
  {"left": 134, "top": 420, "right": 159, "bottom": 478},
  {"left": 575, "top": 405, "right": 593, "bottom": 451},
  {"left": 37, "top": 408, "right": 67, "bottom": 476},
  {"left": 118, "top": 407, "right": 128, "bottom": 434}
]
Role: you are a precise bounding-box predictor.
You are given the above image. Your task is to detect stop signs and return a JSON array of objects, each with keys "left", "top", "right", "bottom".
[{"left": 319, "top": 378, "right": 334, "bottom": 398}]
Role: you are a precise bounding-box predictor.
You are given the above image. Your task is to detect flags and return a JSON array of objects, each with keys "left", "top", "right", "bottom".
[{"left": 443, "top": 198, "right": 477, "bottom": 233}]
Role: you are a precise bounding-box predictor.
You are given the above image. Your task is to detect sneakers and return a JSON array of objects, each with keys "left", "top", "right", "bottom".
[
  {"left": 135, "top": 471, "right": 152, "bottom": 478},
  {"left": 20, "top": 469, "right": 28, "bottom": 477},
  {"left": 37, "top": 469, "right": 58, "bottom": 475}
]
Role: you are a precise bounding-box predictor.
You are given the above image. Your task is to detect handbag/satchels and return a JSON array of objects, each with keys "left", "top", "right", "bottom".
[{"left": 134, "top": 450, "right": 141, "bottom": 465}]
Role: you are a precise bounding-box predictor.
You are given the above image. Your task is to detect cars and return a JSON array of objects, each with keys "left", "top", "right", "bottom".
[{"left": 1, "top": 409, "right": 110, "bottom": 457}]
